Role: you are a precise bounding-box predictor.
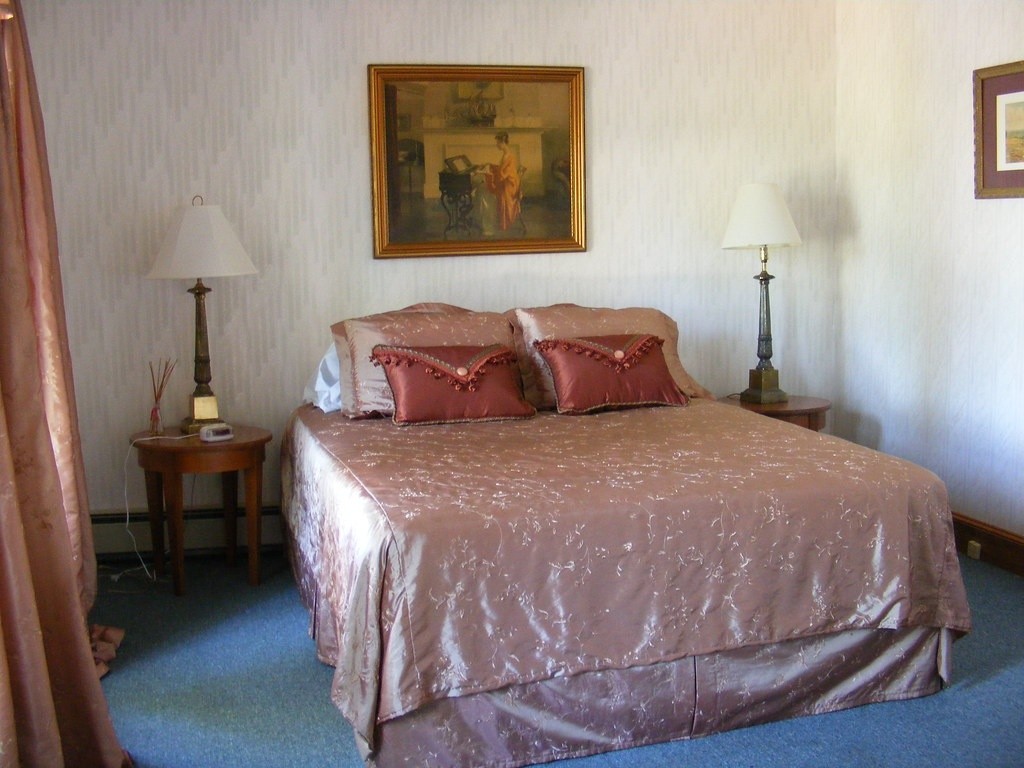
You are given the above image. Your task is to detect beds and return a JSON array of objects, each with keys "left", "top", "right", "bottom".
[{"left": 276, "top": 403, "right": 972, "bottom": 768}]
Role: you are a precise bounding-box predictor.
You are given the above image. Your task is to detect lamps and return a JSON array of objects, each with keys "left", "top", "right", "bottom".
[
  {"left": 144, "top": 194, "right": 259, "bottom": 434},
  {"left": 721, "top": 182, "right": 801, "bottom": 405}
]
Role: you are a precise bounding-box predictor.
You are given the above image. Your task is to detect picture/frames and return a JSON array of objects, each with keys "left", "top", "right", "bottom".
[
  {"left": 972, "top": 61, "right": 1024, "bottom": 200},
  {"left": 368, "top": 64, "right": 586, "bottom": 260}
]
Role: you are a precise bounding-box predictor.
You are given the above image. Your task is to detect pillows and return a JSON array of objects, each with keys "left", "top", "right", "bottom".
[
  {"left": 532, "top": 334, "right": 692, "bottom": 416},
  {"left": 330, "top": 302, "right": 525, "bottom": 420},
  {"left": 502, "top": 303, "right": 717, "bottom": 411},
  {"left": 368, "top": 343, "right": 538, "bottom": 426}
]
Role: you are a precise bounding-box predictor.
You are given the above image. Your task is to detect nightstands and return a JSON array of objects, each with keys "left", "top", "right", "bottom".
[
  {"left": 129, "top": 424, "right": 273, "bottom": 596},
  {"left": 718, "top": 395, "right": 832, "bottom": 433}
]
